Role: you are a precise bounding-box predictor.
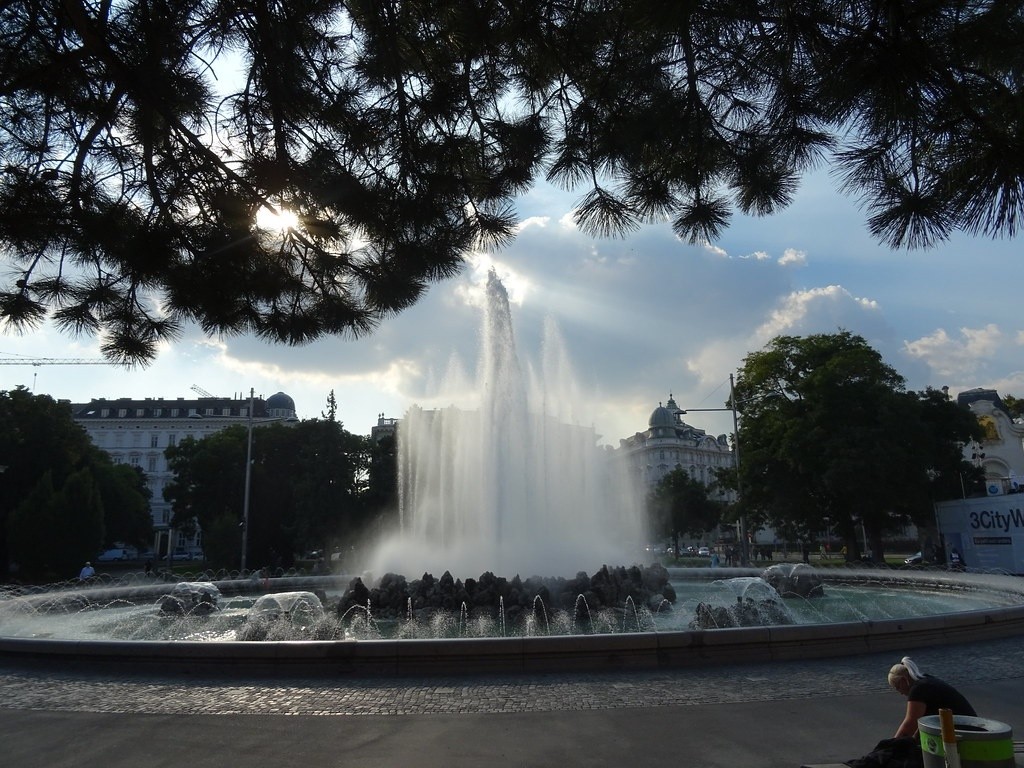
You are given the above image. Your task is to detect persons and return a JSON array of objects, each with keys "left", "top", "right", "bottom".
[
  {"left": 888, "top": 654, "right": 978, "bottom": 744},
  {"left": 801, "top": 542, "right": 848, "bottom": 564},
  {"left": 710, "top": 544, "right": 774, "bottom": 567},
  {"left": 144, "top": 555, "right": 161, "bottom": 578},
  {"left": 79, "top": 561, "right": 96, "bottom": 582}
]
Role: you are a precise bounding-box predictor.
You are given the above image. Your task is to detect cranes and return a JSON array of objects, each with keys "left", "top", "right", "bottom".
[{"left": 0, "top": 351, "right": 132, "bottom": 366}]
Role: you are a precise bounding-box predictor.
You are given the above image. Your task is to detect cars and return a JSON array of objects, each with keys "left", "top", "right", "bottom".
[
  {"left": 162, "top": 550, "right": 191, "bottom": 561},
  {"left": 667, "top": 547, "right": 696, "bottom": 556},
  {"left": 193, "top": 552, "right": 204, "bottom": 561},
  {"left": 646, "top": 545, "right": 666, "bottom": 555},
  {"left": 699, "top": 547, "right": 710, "bottom": 556},
  {"left": 905, "top": 551, "right": 921, "bottom": 564}
]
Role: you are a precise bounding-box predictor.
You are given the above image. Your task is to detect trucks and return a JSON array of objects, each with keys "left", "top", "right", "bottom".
[{"left": 97, "top": 548, "right": 128, "bottom": 561}]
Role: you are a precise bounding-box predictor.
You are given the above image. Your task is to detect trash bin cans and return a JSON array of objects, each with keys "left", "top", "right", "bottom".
[{"left": 916, "top": 714, "right": 1014, "bottom": 768}]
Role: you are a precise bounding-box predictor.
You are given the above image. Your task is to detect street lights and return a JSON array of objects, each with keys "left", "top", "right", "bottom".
[
  {"left": 673, "top": 392, "right": 780, "bottom": 565},
  {"left": 188, "top": 414, "right": 299, "bottom": 577},
  {"left": 851, "top": 516, "right": 867, "bottom": 553}
]
